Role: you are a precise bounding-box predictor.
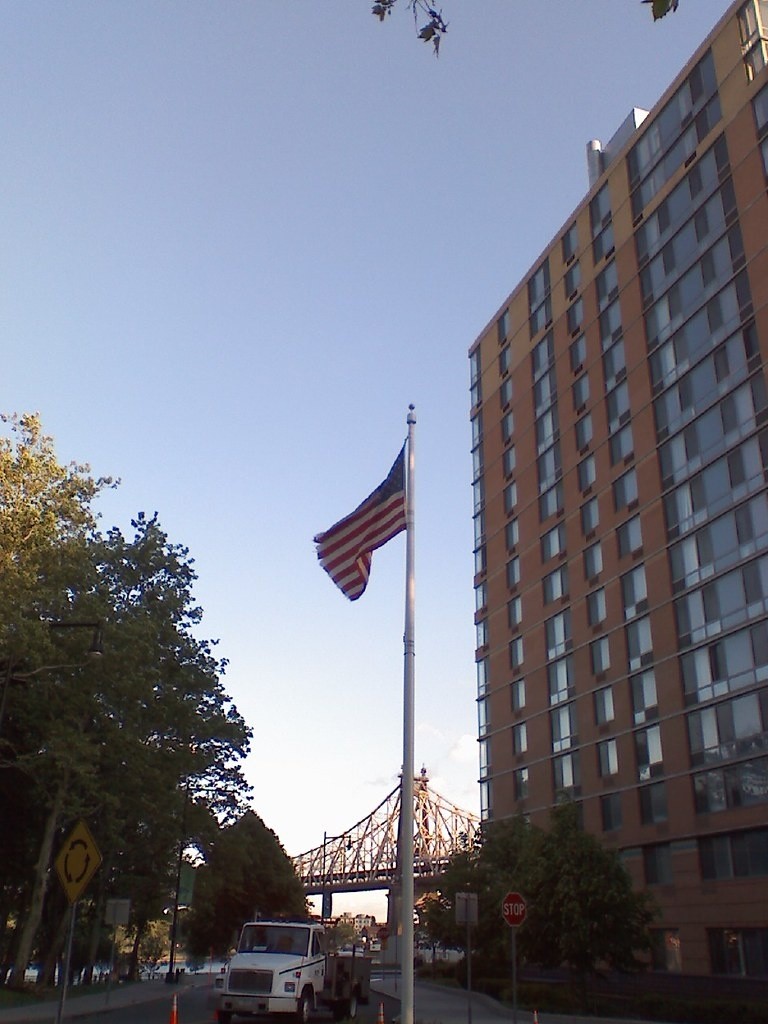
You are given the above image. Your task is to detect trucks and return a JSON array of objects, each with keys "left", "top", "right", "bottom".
[{"left": 214, "top": 919, "right": 372, "bottom": 1024}]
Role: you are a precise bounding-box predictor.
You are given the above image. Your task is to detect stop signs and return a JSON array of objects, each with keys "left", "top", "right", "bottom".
[
  {"left": 378, "top": 928, "right": 390, "bottom": 939},
  {"left": 502, "top": 892, "right": 528, "bottom": 927}
]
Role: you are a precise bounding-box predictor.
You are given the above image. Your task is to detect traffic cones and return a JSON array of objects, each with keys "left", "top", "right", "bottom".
[
  {"left": 170, "top": 993, "right": 179, "bottom": 1024},
  {"left": 532, "top": 1010, "right": 539, "bottom": 1024},
  {"left": 376, "top": 1003, "right": 385, "bottom": 1024}
]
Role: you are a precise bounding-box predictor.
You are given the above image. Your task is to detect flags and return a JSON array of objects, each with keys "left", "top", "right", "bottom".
[{"left": 315, "top": 442, "right": 416, "bottom": 603}]
